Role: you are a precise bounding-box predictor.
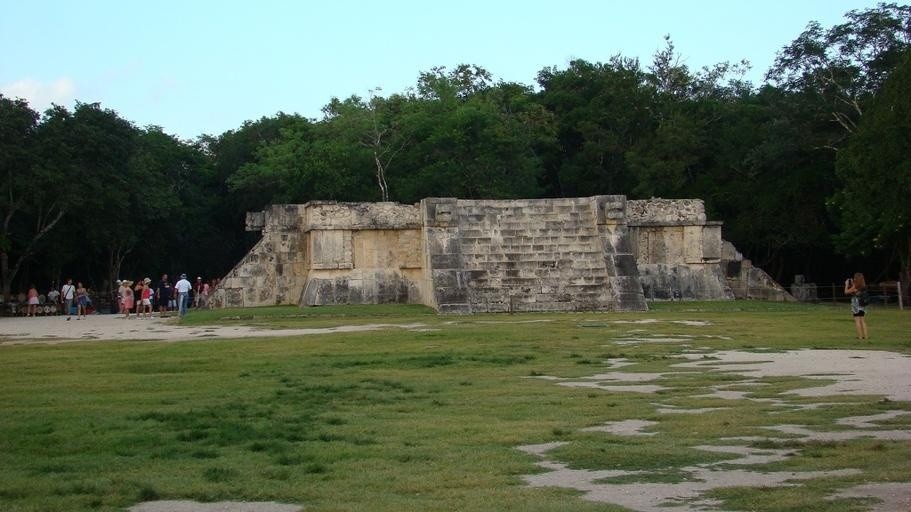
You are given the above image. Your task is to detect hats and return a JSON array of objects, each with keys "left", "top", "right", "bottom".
[
  {"left": 143, "top": 278, "right": 151, "bottom": 283},
  {"left": 180, "top": 273, "right": 186, "bottom": 279},
  {"left": 121, "top": 280, "right": 128, "bottom": 284},
  {"left": 196, "top": 277, "right": 201, "bottom": 280},
  {"left": 115, "top": 279, "right": 121, "bottom": 283}
]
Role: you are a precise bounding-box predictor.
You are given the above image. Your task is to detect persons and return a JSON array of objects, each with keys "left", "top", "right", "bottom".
[
  {"left": 0, "top": 271, "right": 220, "bottom": 322},
  {"left": 844, "top": 272, "right": 868, "bottom": 339}
]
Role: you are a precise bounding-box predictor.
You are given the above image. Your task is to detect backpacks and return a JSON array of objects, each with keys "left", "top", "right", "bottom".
[{"left": 858, "top": 289, "right": 870, "bottom": 307}]
